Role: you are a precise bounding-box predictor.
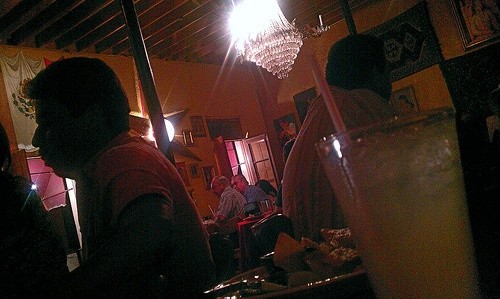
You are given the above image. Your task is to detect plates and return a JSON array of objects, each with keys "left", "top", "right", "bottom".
[
  {"left": 203, "top": 264, "right": 366, "bottom": 298},
  {"left": 243, "top": 212, "right": 263, "bottom": 222}
]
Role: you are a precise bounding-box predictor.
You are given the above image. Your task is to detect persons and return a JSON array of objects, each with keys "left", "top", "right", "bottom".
[
  {"left": 282, "top": 34, "right": 401, "bottom": 242},
  {"left": 0, "top": 58, "right": 219, "bottom": 299},
  {"left": 203, "top": 174, "right": 247, "bottom": 248},
  {"left": 279, "top": 120, "right": 296, "bottom": 138},
  {"left": 275, "top": 140, "right": 295, "bottom": 213},
  {"left": 255, "top": 180, "right": 277, "bottom": 201},
  {"left": 230, "top": 174, "right": 268, "bottom": 218}
]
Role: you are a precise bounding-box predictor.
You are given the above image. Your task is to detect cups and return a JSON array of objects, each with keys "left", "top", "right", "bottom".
[
  {"left": 258, "top": 199, "right": 274, "bottom": 217},
  {"left": 210, "top": 209, "right": 219, "bottom": 221},
  {"left": 314, "top": 106, "right": 483, "bottom": 299},
  {"left": 259, "top": 246, "right": 317, "bottom": 284}
]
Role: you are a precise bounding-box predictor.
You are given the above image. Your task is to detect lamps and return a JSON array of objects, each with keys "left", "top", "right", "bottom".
[{"left": 235, "top": 0, "right": 331, "bottom": 80}]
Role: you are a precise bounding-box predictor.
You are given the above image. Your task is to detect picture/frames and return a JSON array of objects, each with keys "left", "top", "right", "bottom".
[
  {"left": 273, "top": 112, "right": 299, "bottom": 147},
  {"left": 445, "top": 0, "right": 500, "bottom": 52},
  {"left": 181, "top": 116, "right": 207, "bottom": 146},
  {"left": 389, "top": 84, "right": 420, "bottom": 116},
  {"left": 176, "top": 161, "right": 217, "bottom": 191}
]
